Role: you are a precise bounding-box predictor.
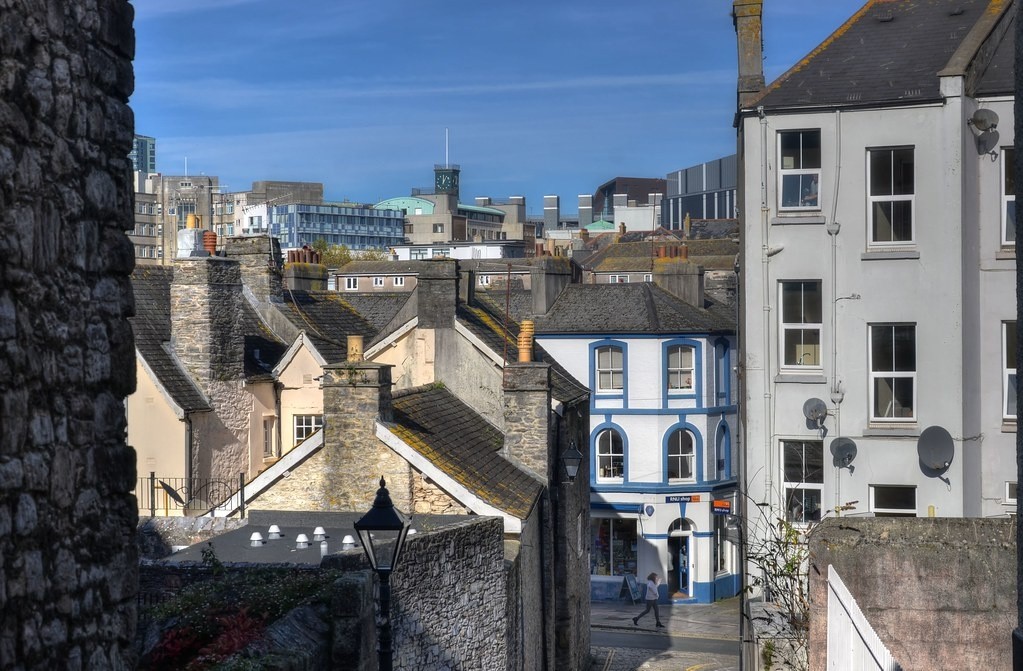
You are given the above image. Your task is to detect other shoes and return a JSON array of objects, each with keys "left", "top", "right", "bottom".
[
  {"left": 656, "top": 622, "right": 665, "bottom": 627},
  {"left": 633, "top": 617, "right": 638, "bottom": 626}
]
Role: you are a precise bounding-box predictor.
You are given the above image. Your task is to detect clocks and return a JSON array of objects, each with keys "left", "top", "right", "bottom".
[
  {"left": 436, "top": 174, "right": 450, "bottom": 189},
  {"left": 452, "top": 175, "right": 460, "bottom": 188}
]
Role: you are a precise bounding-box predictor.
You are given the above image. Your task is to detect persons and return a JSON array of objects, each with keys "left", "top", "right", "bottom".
[
  {"left": 633, "top": 573, "right": 666, "bottom": 628},
  {"left": 668, "top": 552, "right": 674, "bottom": 590}
]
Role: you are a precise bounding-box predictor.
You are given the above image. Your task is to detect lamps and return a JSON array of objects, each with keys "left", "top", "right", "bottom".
[
  {"left": 354, "top": 472, "right": 414, "bottom": 671},
  {"left": 557, "top": 438, "right": 583, "bottom": 485}
]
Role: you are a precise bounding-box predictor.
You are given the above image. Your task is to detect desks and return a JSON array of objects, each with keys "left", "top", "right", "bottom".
[{"left": 606, "top": 467, "right": 618, "bottom": 477}]
